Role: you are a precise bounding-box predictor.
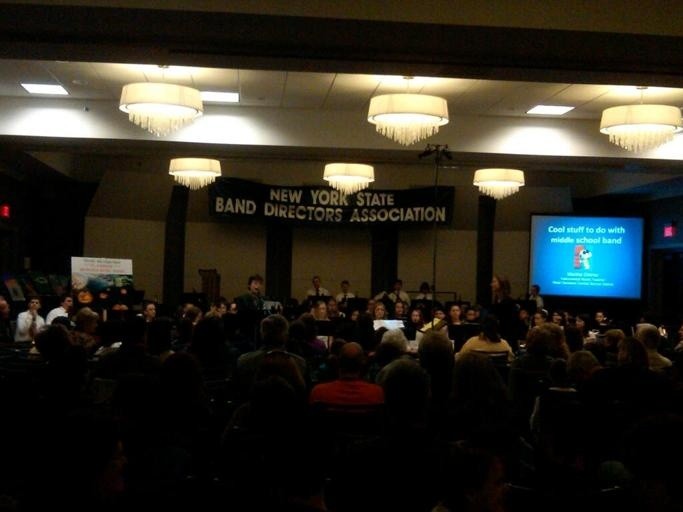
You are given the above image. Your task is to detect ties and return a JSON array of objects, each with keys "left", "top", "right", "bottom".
[
  {"left": 316, "top": 289, "right": 319, "bottom": 296},
  {"left": 344, "top": 294, "right": 347, "bottom": 302}
]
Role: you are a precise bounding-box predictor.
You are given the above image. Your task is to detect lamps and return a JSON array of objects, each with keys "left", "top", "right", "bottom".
[
  {"left": 472, "top": 168, "right": 527, "bottom": 200},
  {"left": 599, "top": 90, "right": 682, "bottom": 152},
  {"left": 323, "top": 163, "right": 377, "bottom": 196},
  {"left": 168, "top": 157, "right": 222, "bottom": 191},
  {"left": 119, "top": 82, "right": 205, "bottom": 138},
  {"left": 366, "top": 93, "right": 451, "bottom": 146}
]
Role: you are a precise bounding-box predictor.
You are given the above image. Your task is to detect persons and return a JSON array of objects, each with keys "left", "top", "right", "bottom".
[{"left": 1, "top": 273, "right": 681, "bottom": 511}]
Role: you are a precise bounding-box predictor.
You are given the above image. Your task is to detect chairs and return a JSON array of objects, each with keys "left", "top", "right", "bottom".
[{"left": 1, "top": 319, "right": 682, "bottom": 510}]
